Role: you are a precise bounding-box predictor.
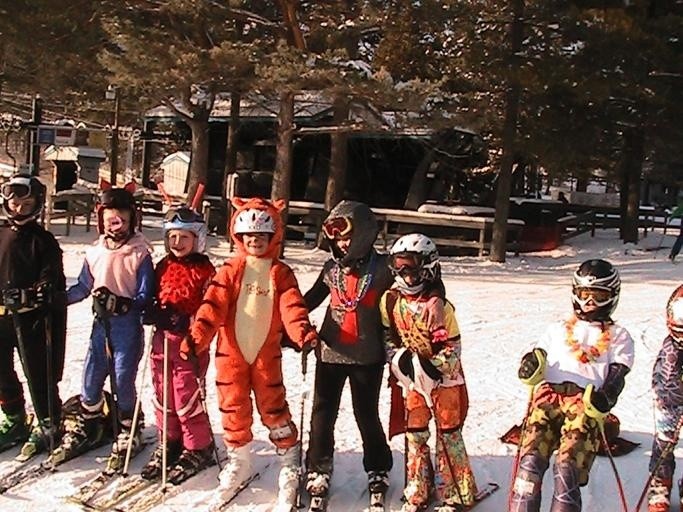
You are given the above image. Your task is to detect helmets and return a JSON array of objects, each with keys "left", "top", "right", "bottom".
[
  {"left": 571, "top": 260, "right": 683, "bottom": 348},
  {"left": 232, "top": 209, "right": 276, "bottom": 236},
  {"left": 98, "top": 187, "right": 138, "bottom": 225},
  {"left": 389, "top": 233, "right": 439, "bottom": 282},
  {"left": 322, "top": 200, "right": 374, "bottom": 239},
  {"left": 2, "top": 177, "right": 47, "bottom": 200},
  {"left": 164, "top": 205, "right": 206, "bottom": 254}
]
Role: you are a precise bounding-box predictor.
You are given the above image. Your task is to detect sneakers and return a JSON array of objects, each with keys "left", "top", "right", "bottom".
[
  {"left": 277, "top": 439, "right": 300, "bottom": 490},
  {"left": 142, "top": 439, "right": 186, "bottom": 478},
  {"left": 168, "top": 442, "right": 215, "bottom": 480},
  {"left": 306, "top": 465, "right": 334, "bottom": 495},
  {"left": 1, "top": 405, "right": 144, "bottom": 456},
  {"left": 366, "top": 468, "right": 390, "bottom": 493},
  {"left": 219, "top": 441, "right": 251, "bottom": 491}
]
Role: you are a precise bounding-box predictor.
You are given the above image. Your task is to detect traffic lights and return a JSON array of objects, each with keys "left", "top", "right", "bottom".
[{"left": 33, "top": 97, "right": 44, "bottom": 126}]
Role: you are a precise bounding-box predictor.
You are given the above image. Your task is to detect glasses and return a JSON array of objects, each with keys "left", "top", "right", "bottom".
[{"left": 164, "top": 207, "right": 205, "bottom": 223}]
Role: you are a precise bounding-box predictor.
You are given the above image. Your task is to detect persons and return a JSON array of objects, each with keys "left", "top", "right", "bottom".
[
  {"left": 178, "top": 196, "right": 318, "bottom": 511},
  {"left": 39, "top": 179, "right": 155, "bottom": 473},
  {"left": 377, "top": 232, "right": 479, "bottom": 511},
  {"left": 140, "top": 207, "right": 217, "bottom": 484},
  {"left": 507, "top": 259, "right": 635, "bottom": 512},
  {"left": 280, "top": 199, "right": 406, "bottom": 512},
  {"left": 0, "top": 173, "right": 67, "bottom": 462},
  {"left": 645, "top": 284, "right": 683, "bottom": 511}
]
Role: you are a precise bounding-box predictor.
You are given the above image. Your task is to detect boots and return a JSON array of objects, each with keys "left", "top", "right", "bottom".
[{"left": 647, "top": 472, "right": 672, "bottom": 511}]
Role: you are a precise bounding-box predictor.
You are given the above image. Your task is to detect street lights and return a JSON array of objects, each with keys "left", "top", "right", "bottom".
[{"left": 104, "top": 81, "right": 122, "bottom": 185}]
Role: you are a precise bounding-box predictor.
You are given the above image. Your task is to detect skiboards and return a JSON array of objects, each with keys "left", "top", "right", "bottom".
[
  {"left": 0, "top": 425, "right": 155, "bottom": 502},
  {"left": 403, "top": 481, "right": 498, "bottom": 512},
  {"left": 208, "top": 458, "right": 304, "bottom": 512},
  {"left": 79, "top": 444, "right": 231, "bottom": 512},
  {"left": 310, "top": 494, "right": 384, "bottom": 512}
]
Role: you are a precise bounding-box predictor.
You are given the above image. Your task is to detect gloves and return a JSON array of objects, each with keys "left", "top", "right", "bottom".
[
  {"left": 390, "top": 347, "right": 444, "bottom": 394},
  {"left": 518, "top": 348, "right": 547, "bottom": 385},
  {"left": 94, "top": 286, "right": 133, "bottom": 314},
  {"left": 142, "top": 299, "right": 191, "bottom": 335},
  {"left": 2, "top": 287, "right": 46, "bottom": 308}
]
